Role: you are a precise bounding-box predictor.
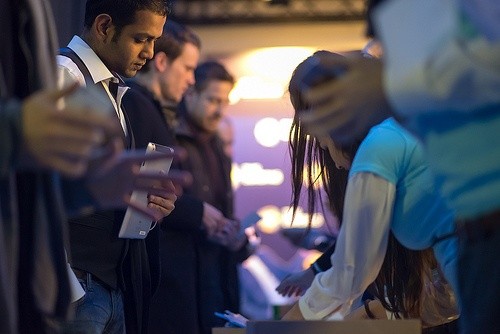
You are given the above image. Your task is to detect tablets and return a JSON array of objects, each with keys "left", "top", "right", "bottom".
[{"left": 119, "top": 143, "right": 174, "bottom": 239}]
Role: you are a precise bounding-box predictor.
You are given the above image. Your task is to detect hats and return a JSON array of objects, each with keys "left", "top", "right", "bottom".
[{"left": 289, "top": 50, "right": 363, "bottom": 133}]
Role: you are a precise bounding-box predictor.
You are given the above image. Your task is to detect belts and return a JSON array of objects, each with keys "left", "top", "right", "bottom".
[{"left": 71, "top": 267, "right": 97, "bottom": 280}]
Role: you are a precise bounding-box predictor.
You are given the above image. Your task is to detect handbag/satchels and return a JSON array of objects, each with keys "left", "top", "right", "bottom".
[{"left": 401, "top": 248, "right": 461, "bottom": 329}]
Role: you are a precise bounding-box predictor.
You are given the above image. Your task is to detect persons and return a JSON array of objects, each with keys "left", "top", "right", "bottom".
[
  {"left": 274, "top": 0, "right": 499, "bottom": 334},
  {"left": 0, "top": 0, "right": 248, "bottom": 334}
]
